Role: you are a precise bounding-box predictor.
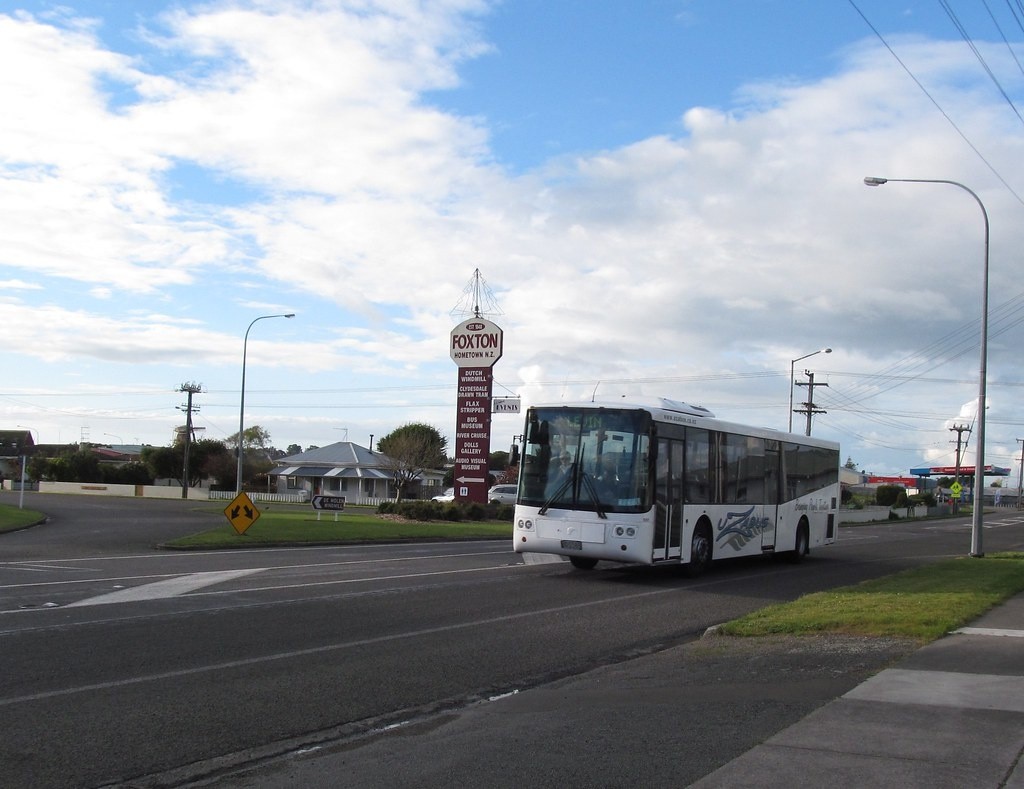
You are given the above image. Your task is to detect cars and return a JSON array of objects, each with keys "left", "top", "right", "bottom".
[{"left": 430, "top": 486, "right": 454, "bottom": 504}]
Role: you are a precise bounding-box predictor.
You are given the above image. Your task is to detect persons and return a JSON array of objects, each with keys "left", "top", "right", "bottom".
[
  {"left": 949, "top": 498, "right": 953, "bottom": 514},
  {"left": 555, "top": 451, "right": 574, "bottom": 483},
  {"left": 958, "top": 499, "right": 961, "bottom": 506},
  {"left": 603, "top": 469, "right": 614, "bottom": 485}
]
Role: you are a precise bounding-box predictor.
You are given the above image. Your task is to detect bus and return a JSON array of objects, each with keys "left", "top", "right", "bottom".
[{"left": 512, "top": 393, "right": 840, "bottom": 578}]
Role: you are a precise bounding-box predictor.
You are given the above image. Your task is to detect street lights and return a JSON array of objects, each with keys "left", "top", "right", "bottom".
[
  {"left": 789, "top": 348, "right": 832, "bottom": 434},
  {"left": 866, "top": 175, "right": 990, "bottom": 559},
  {"left": 235, "top": 313, "right": 296, "bottom": 496}
]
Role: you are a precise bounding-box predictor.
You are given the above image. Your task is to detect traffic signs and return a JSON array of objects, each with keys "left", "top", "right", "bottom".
[{"left": 311, "top": 495, "right": 346, "bottom": 512}]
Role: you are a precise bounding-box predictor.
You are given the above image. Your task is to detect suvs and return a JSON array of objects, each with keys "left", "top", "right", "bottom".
[{"left": 488, "top": 484, "right": 517, "bottom": 508}]
